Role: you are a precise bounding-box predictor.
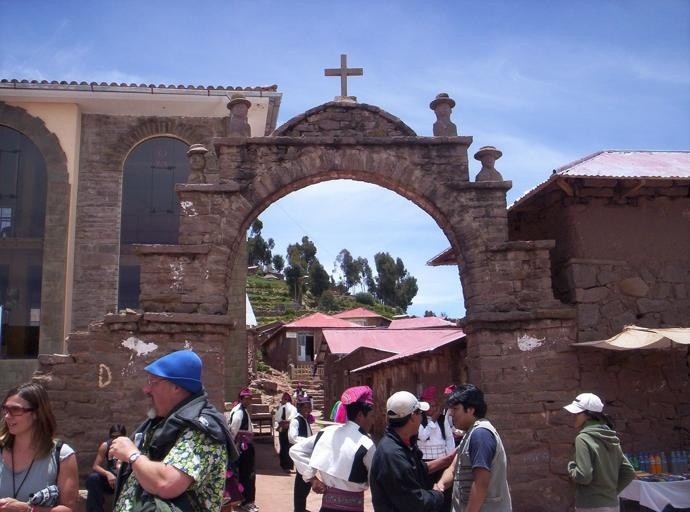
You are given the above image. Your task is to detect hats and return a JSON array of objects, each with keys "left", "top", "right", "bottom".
[
  {"left": 387, "top": 390, "right": 430, "bottom": 419},
  {"left": 562, "top": 392, "right": 605, "bottom": 414},
  {"left": 282, "top": 392, "right": 292, "bottom": 402},
  {"left": 297, "top": 396, "right": 311, "bottom": 403},
  {"left": 422, "top": 385, "right": 438, "bottom": 401},
  {"left": 143, "top": 349, "right": 203, "bottom": 394},
  {"left": 238, "top": 387, "right": 253, "bottom": 396},
  {"left": 341, "top": 386, "right": 374, "bottom": 407}
]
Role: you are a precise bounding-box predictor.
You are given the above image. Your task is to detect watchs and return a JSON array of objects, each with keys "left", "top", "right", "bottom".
[{"left": 127, "top": 451, "right": 141, "bottom": 465}]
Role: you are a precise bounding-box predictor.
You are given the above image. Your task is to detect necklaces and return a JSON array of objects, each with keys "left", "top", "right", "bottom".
[{"left": 11, "top": 443, "right": 38, "bottom": 499}]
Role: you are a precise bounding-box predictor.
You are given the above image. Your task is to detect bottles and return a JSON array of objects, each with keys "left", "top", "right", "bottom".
[
  {"left": 639, "top": 451, "right": 667, "bottom": 474},
  {"left": 670, "top": 450, "right": 688, "bottom": 473}
]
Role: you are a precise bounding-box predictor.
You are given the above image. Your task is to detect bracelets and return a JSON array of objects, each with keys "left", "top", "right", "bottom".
[{"left": 24, "top": 504, "right": 37, "bottom": 512}]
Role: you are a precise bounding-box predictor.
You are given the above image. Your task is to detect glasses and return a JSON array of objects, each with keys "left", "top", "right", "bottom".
[
  {"left": 146, "top": 375, "right": 169, "bottom": 387},
  {"left": 2, "top": 404, "right": 35, "bottom": 416}
]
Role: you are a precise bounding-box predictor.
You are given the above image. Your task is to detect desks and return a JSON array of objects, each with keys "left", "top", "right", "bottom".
[
  {"left": 620, "top": 473, "right": 690, "bottom": 511},
  {"left": 251, "top": 413, "right": 274, "bottom": 443}
]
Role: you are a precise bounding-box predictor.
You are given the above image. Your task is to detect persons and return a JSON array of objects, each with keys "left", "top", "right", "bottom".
[
  {"left": 370, "top": 390, "right": 457, "bottom": 512},
  {"left": 563, "top": 393, "right": 637, "bottom": 511},
  {"left": 442, "top": 384, "right": 466, "bottom": 448},
  {"left": 417, "top": 386, "right": 456, "bottom": 512},
  {"left": 288, "top": 385, "right": 376, "bottom": 512},
  {"left": 227, "top": 383, "right": 315, "bottom": 512},
  {"left": 0, "top": 381, "right": 79, "bottom": 512},
  {"left": 426, "top": 383, "right": 512, "bottom": 512},
  {"left": 108, "top": 351, "right": 240, "bottom": 512},
  {"left": 85, "top": 424, "right": 126, "bottom": 511}
]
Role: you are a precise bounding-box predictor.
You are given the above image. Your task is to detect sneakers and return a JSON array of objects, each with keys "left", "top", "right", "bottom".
[{"left": 242, "top": 503, "right": 258, "bottom": 512}]
